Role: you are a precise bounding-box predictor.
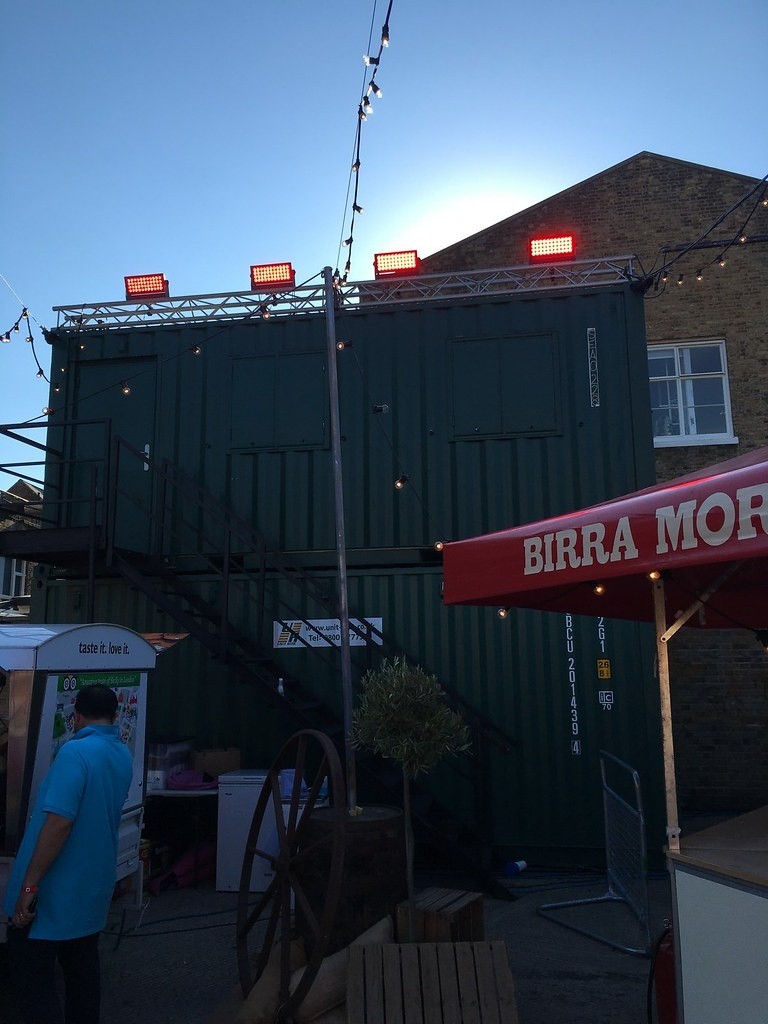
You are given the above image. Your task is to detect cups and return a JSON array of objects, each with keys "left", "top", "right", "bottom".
[{"left": 504, "top": 860, "right": 527, "bottom": 877}]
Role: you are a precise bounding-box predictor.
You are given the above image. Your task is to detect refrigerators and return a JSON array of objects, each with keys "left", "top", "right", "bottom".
[{"left": 215, "top": 767, "right": 330, "bottom": 893}]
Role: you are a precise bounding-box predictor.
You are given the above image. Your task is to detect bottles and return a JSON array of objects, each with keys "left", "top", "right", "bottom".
[{"left": 277, "top": 678, "right": 284, "bottom": 696}]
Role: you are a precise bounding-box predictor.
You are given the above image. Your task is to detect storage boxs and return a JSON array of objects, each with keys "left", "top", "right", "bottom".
[{"left": 192, "top": 746, "right": 241, "bottom": 779}]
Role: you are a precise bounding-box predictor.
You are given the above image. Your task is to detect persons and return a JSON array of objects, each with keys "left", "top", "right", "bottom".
[{"left": 0, "top": 684, "right": 133, "bottom": 1024}]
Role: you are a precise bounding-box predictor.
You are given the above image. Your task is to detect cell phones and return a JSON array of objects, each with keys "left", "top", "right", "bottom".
[{"left": 28, "top": 896, "right": 37, "bottom": 913}]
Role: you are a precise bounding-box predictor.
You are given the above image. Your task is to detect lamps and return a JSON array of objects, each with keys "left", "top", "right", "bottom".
[
  {"left": 250, "top": 263, "right": 296, "bottom": 291},
  {"left": 373, "top": 250, "right": 422, "bottom": 280},
  {"left": 528, "top": 230, "right": 577, "bottom": 264},
  {"left": 124, "top": 274, "right": 169, "bottom": 300}
]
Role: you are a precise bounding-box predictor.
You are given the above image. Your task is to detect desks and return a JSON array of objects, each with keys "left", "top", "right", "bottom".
[{"left": 146, "top": 790, "right": 218, "bottom": 826}]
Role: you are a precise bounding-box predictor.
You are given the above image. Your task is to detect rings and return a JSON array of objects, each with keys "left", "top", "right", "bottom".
[{"left": 19, "top": 912, "right": 23, "bottom": 920}]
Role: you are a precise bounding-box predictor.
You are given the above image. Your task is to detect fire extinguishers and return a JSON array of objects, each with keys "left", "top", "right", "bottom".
[{"left": 652, "top": 917, "right": 677, "bottom": 1024}]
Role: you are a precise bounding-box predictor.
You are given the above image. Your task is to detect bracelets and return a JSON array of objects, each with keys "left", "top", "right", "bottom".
[{"left": 22, "top": 886, "right": 39, "bottom": 893}]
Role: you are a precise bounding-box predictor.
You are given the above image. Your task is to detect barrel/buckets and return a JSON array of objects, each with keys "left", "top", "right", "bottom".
[{"left": 125, "top": 838, "right": 150, "bottom": 889}]
